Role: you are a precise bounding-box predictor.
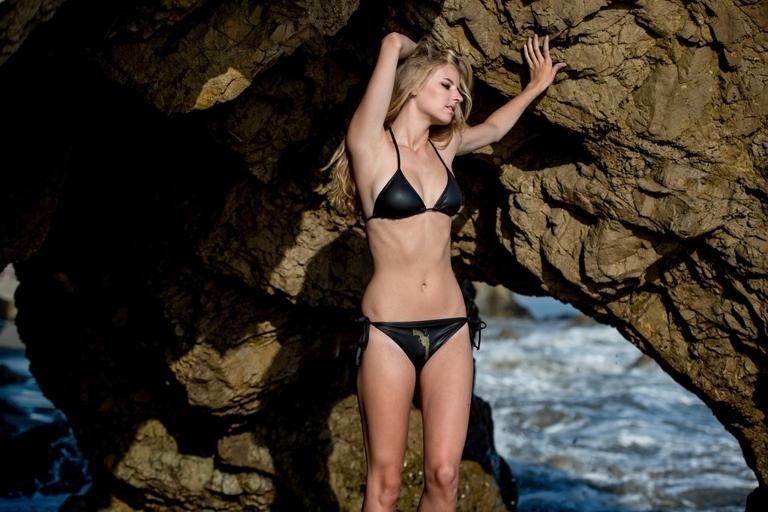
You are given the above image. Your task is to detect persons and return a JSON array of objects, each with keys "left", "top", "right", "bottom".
[{"left": 345, "top": 30, "right": 569, "bottom": 512}]
